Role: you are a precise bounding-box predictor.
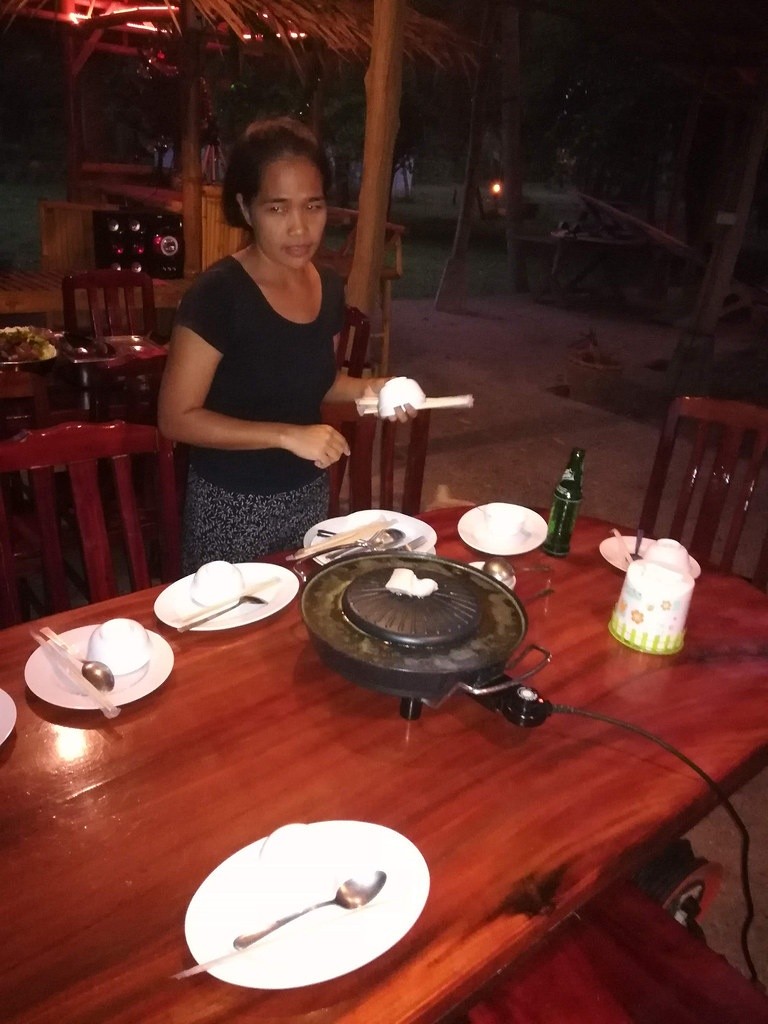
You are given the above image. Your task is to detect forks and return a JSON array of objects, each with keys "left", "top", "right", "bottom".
[
  {"left": 298, "top": 529, "right": 384, "bottom": 564},
  {"left": 343, "top": 535, "right": 428, "bottom": 557}
]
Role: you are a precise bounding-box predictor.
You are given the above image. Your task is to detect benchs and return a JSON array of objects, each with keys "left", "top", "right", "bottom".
[
  {"left": 0, "top": 478, "right": 189, "bottom": 576},
  {"left": 0, "top": 266, "right": 182, "bottom": 314}
]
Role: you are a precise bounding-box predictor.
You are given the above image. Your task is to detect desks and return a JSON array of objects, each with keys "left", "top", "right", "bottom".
[
  {"left": 516, "top": 223, "right": 655, "bottom": 304},
  {"left": 0, "top": 505, "right": 768, "bottom": 1023},
  {"left": 0, "top": 332, "right": 165, "bottom": 437}
]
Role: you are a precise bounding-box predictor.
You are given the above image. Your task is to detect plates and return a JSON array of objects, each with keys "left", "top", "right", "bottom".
[
  {"left": 154, "top": 563, "right": 300, "bottom": 631},
  {"left": 0, "top": 688, "right": 17, "bottom": 745},
  {"left": 458, "top": 502, "right": 548, "bottom": 556},
  {"left": 25, "top": 625, "right": 175, "bottom": 710},
  {"left": 0, "top": 344, "right": 58, "bottom": 365},
  {"left": 184, "top": 821, "right": 431, "bottom": 989},
  {"left": 599, "top": 536, "right": 701, "bottom": 580},
  {"left": 303, "top": 510, "right": 437, "bottom": 567},
  {"left": 469, "top": 561, "right": 516, "bottom": 592}
]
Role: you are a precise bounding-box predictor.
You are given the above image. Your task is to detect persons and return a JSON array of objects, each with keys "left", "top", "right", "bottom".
[{"left": 156, "top": 116, "right": 418, "bottom": 573}]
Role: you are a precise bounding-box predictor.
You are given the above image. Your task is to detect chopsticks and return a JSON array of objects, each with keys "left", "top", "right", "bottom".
[
  {"left": 613, "top": 529, "right": 634, "bottom": 564},
  {"left": 360, "top": 394, "right": 475, "bottom": 414},
  {"left": 33, "top": 634, "right": 115, "bottom": 712},
  {"left": 176, "top": 577, "right": 281, "bottom": 623},
  {"left": 286, "top": 516, "right": 383, "bottom": 561}
]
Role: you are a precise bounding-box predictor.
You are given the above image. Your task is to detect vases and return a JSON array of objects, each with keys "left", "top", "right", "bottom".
[{"left": 565, "top": 349, "right": 624, "bottom": 405}]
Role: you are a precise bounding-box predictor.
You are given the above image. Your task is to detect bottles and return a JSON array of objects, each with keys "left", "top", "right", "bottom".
[{"left": 543, "top": 448, "right": 586, "bottom": 556}]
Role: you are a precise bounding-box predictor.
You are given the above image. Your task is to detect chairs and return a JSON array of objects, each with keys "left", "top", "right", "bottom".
[
  {"left": 325, "top": 406, "right": 429, "bottom": 518},
  {"left": 640, "top": 397, "right": 768, "bottom": 590},
  {"left": 331, "top": 305, "right": 374, "bottom": 510},
  {"left": 0, "top": 420, "right": 190, "bottom": 628},
  {"left": 62, "top": 268, "right": 159, "bottom": 336}
]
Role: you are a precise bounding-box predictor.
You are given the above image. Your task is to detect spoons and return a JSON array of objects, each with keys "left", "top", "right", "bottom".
[
  {"left": 177, "top": 595, "right": 269, "bottom": 633},
  {"left": 625, "top": 529, "right": 644, "bottom": 562},
  {"left": 329, "top": 529, "right": 405, "bottom": 561},
  {"left": 40, "top": 627, "right": 115, "bottom": 692},
  {"left": 233, "top": 870, "right": 387, "bottom": 950},
  {"left": 484, "top": 557, "right": 519, "bottom": 582}
]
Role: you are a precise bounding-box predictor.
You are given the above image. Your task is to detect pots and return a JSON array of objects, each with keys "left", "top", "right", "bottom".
[{"left": 294, "top": 545, "right": 552, "bottom": 733}]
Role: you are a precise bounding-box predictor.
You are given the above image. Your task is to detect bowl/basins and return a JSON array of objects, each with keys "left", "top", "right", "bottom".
[
  {"left": 87, "top": 619, "right": 152, "bottom": 675},
  {"left": 192, "top": 561, "right": 244, "bottom": 606},
  {"left": 378, "top": 376, "right": 426, "bottom": 416}
]
